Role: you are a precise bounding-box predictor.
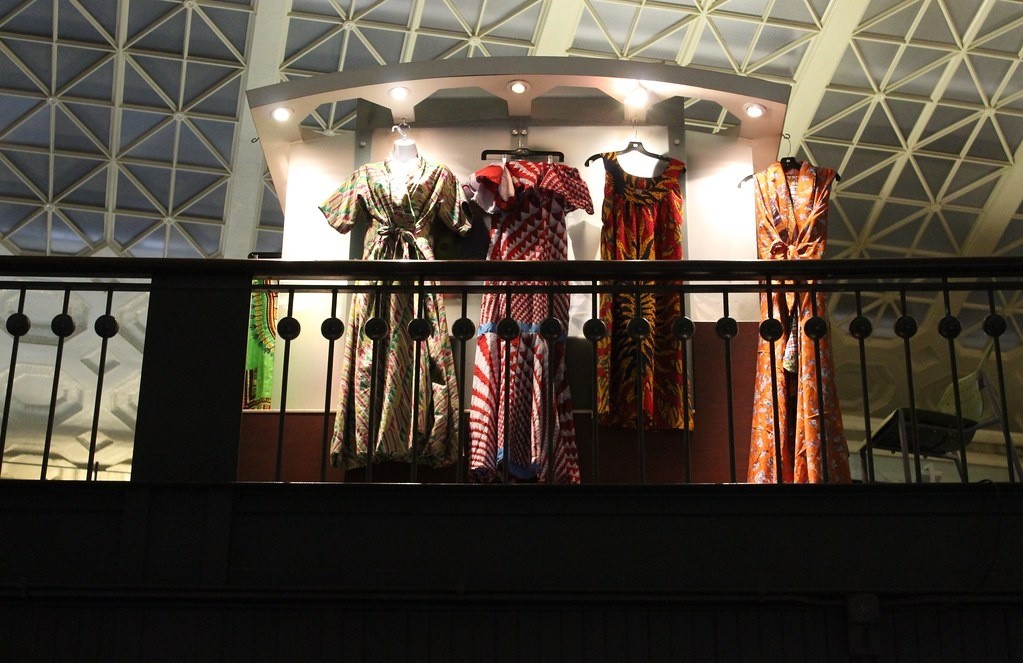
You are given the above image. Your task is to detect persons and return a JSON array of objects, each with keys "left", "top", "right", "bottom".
[{"left": 320, "top": 138, "right": 474, "bottom": 477}]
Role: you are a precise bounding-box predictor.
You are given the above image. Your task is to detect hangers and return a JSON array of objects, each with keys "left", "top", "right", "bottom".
[
  {"left": 500, "top": 152, "right": 553, "bottom": 165},
  {"left": 584, "top": 118, "right": 687, "bottom": 173},
  {"left": 737, "top": 136, "right": 842, "bottom": 182}
]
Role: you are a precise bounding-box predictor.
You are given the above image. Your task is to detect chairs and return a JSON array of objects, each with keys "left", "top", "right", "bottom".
[{"left": 857, "top": 367, "right": 983, "bottom": 483}]
